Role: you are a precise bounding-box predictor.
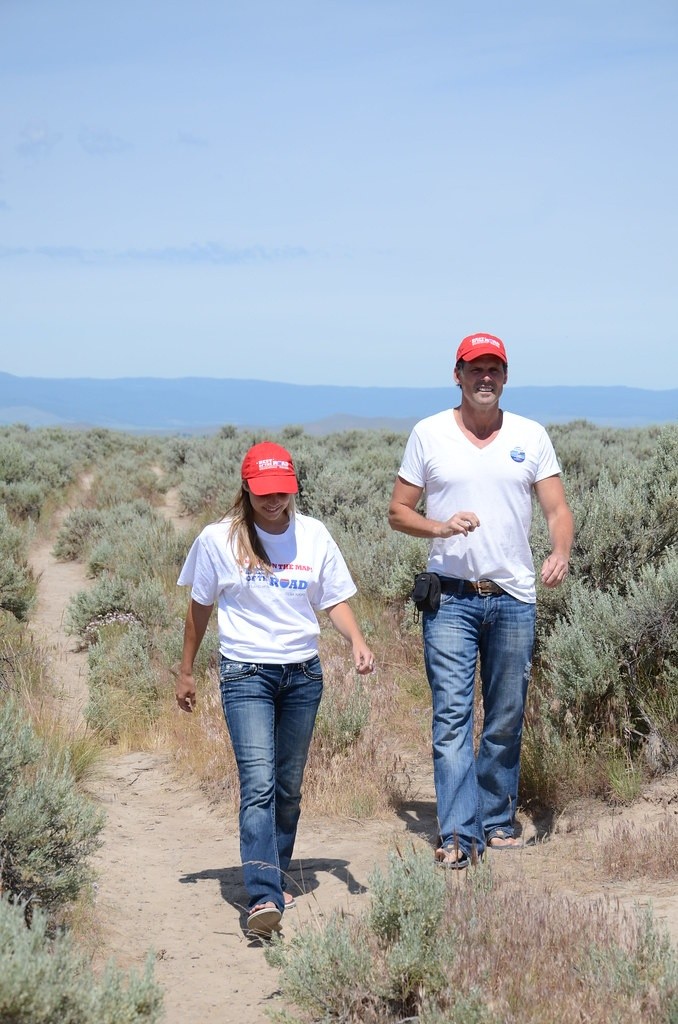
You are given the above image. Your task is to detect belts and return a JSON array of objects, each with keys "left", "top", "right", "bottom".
[{"left": 438, "top": 575, "right": 505, "bottom": 598}]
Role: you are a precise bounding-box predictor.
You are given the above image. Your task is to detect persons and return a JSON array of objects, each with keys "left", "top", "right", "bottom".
[
  {"left": 388, "top": 332, "right": 574, "bottom": 870},
  {"left": 176, "top": 440, "right": 376, "bottom": 930}
]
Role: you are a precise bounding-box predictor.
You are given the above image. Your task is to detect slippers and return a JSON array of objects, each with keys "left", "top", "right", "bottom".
[
  {"left": 247, "top": 898, "right": 296, "bottom": 936},
  {"left": 487, "top": 830, "right": 523, "bottom": 850},
  {"left": 435, "top": 843, "right": 485, "bottom": 868}
]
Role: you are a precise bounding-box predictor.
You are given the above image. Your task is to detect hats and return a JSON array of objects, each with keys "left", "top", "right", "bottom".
[
  {"left": 456, "top": 333, "right": 507, "bottom": 363},
  {"left": 241, "top": 440, "right": 298, "bottom": 496}
]
